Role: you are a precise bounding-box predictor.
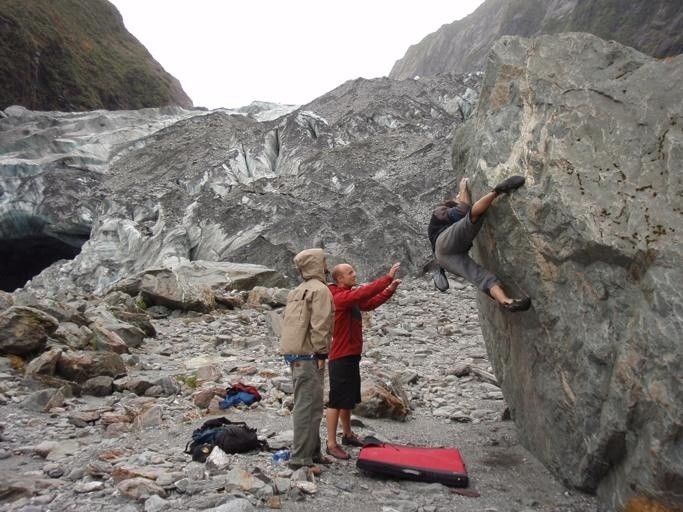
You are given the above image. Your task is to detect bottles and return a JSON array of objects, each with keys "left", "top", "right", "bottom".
[{"left": 273, "top": 450, "right": 292, "bottom": 461}]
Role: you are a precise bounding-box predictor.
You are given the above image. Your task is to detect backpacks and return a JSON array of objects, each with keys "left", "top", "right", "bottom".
[
  {"left": 184, "top": 415, "right": 258, "bottom": 462},
  {"left": 218, "top": 382, "right": 262, "bottom": 410}
]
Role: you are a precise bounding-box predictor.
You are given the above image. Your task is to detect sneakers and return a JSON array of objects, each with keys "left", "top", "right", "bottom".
[
  {"left": 342, "top": 431, "right": 365, "bottom": 447},
  {"left": 311, "top": 452, "right": 333, "bottom": 464},
  {"left": 290, "top": 461, "right": 322, "bottom": 476},
  {"left": 494, "top": 175, "right": 527, "bottom": 196},
  {"left": 325, "top": 444, "right": 351, "bottom": 460},
  {"left": 503, "top": 297, "right": 532, "bottom": 312}
]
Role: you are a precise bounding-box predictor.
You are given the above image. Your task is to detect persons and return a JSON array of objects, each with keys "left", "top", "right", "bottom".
[
  {"left": 425, "top": 174, "right": 531, "bottom": 314},
  {"left": 325, "top": 261, "right": 403, "bottom": 462},
  {"left": 278, "top": 247, "right": 336, "bottom": 479}
]
